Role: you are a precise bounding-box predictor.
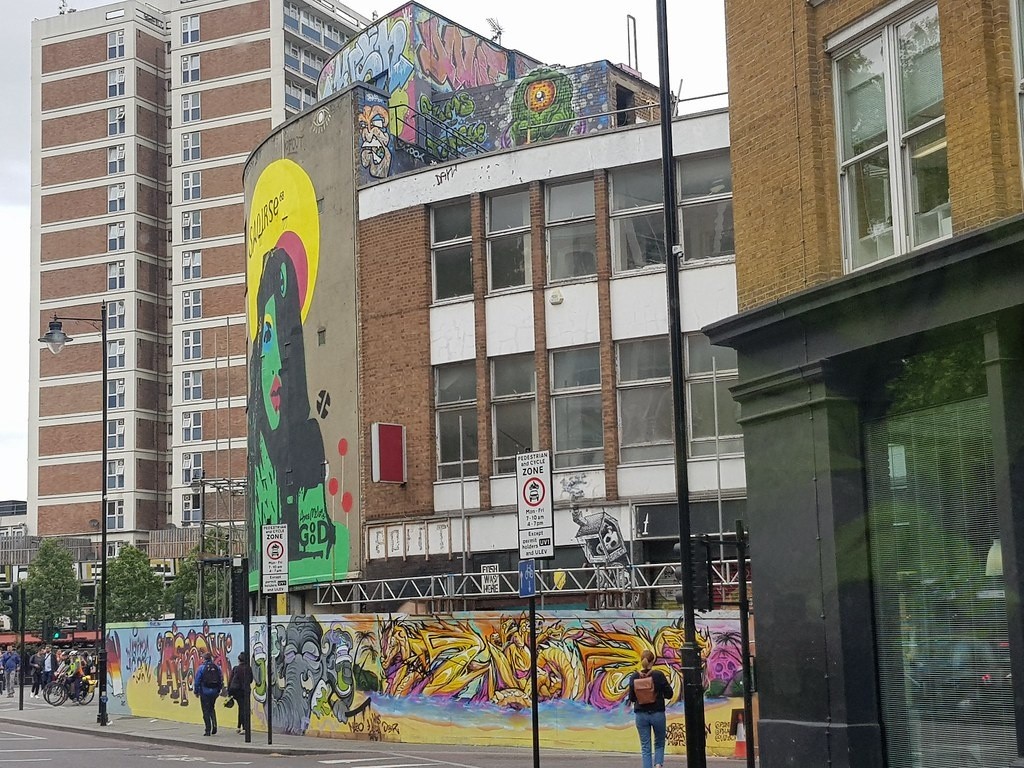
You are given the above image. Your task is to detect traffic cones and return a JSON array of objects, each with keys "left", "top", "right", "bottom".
[{"left": 727, "top": 714, "right": 748, "bottom": 760}]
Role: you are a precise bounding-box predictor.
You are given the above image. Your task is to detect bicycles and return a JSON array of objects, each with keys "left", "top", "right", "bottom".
[{"left": 42, "top": 671, "right": 95, "bottom": 706}]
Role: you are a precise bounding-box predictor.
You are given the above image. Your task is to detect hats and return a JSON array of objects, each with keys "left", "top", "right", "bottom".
[
  {"left": 238, "top": 652, "right": 244, "bottom": 662},
  {"left": 224, "top": 698, "right": 234, "bottom": 708},
  {"left": 201, "top": 652, "right": 211, "bottom": 659}
]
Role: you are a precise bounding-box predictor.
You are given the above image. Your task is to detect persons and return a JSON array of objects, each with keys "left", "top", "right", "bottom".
[
  {"left": 40, "top": 645, "right": 58, "bottom": 694},
  {"left": 29, "top": 647, "right": 44, "bottom": 698},
  {"left": 2, "top": 645, "right": 19, "bottom": 698},
  {"left": 54, "top": 648, "right": 94, "bottom": 706},
  {"left": 194, "top": 653, "right": 223, "bottom": 736},
  {"left": 629, "top": 650, "right": 674, "bottom": 768},
  {"left": 227, "top": 654, "right": 253, "bottom": 735},
  {"left": 0, "top": 649, "right": 6, "bottom": 690}
]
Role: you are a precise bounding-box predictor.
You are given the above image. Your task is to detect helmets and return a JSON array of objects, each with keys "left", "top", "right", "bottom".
[{"left": 69, "top": 651, "right": 78, "bottom": 655}]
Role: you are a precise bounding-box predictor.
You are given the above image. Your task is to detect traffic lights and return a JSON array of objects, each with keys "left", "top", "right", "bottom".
[
  {"left": 53, "top": 627, "right": 67, "bottom": 639},
  {"left": 3, "top": 585, "right": 19, "bottom": 632},
  {"left": 38, "top": 618, "right": 49, "bottom": 640},
  {"left": 675, "top": 534, "right": 708, "bottom": 612}
]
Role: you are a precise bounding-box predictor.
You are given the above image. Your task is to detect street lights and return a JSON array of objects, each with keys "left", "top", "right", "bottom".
[
  {"left": 36, "top": 299, "right": 108, "bottom": 726},
  {"left": 202, "top": 556, "right": 250, "bottom": 744},
  {"left": 187, "top": 467, "right": 207, "bottom": 620}
]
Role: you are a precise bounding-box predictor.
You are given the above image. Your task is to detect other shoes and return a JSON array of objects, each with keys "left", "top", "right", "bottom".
[
  {"left": 35, "top": 695, "right": 39, "bottom": 699},
  {"left": 236, "top": 729, "right": 241, "bottom": 733},
  {"left": 239, "top": 730, "right": 245, "bottom": 734},
  {"left": 212, "top": 728, "right": 217, "bottom": 734},
  {"left": 76, "top": 701, "right": 79, "bottom": 705},
  {"left": 204, "top": 733, "right": 210, "bottom": 736},
  {"left": 7, "top": 694, "right": 9, "bottom": 697},
  {"left": 11, "top": 693, "right": 14, "bottom": 697},
  {"left": 31, "top": 692, "right": 34, "bottom": 698}
]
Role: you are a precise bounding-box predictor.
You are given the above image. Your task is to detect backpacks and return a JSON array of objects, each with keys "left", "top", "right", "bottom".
[
  {"left": 238, "top": 665, "right": 249, "bottom": 681},
  {"left": 204, "top": 662, "right": 221, "bottom": 688},
  {"left": 633, "top": 669, "right": 656, "bottom": 704},
  {"left": 84, "top": 665, "right": 90, "bottom": 675}
]
